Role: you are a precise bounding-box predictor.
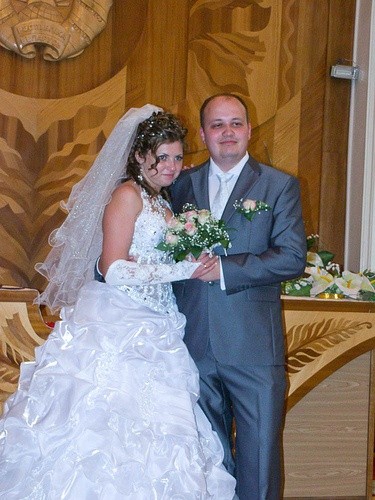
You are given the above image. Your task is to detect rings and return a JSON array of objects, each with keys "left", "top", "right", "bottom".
[{"left": 202, "top": 264, "right": 207, "bottom": 268}]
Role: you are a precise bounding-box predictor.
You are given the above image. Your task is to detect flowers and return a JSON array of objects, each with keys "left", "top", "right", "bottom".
[
  {"left": 154, "top": 203, "right": 238, "bottom": 286},
  {"left": 233, "top": 198, "right": 272, "bottom": 223},
  {"left": 280, "top": 234, "right": 375, "bottom": 300}
]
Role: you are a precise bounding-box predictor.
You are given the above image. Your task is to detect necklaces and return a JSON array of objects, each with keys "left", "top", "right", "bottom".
[{"left": 134, "top": 182, "right": 167, "bottom": 216}]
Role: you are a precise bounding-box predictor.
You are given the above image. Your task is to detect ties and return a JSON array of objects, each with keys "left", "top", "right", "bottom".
[{"left": 211, "top": 173, "right": 235, "bottom": 222}]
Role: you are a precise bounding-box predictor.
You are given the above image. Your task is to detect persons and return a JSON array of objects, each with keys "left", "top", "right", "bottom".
[
  {"left": 94, "top": 93, "right": 308, "bottom": 500},
  {"left": 0, "top": 103, "right": 237, "bottom": 500}
]
